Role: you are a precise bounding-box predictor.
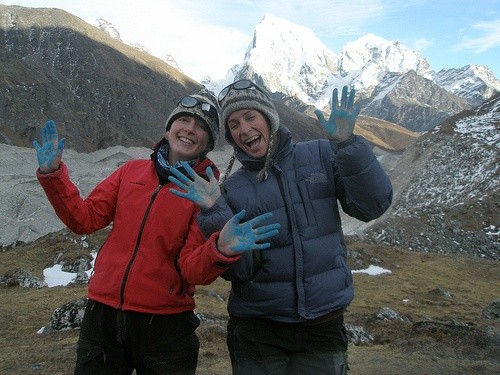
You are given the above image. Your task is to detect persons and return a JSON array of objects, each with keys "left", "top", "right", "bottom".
[
  {"left": 167, "top": 79, "right": 394, "bottom": 375},
  {"left": 31, "top": 90, "right": 281, "bottom": 375}
]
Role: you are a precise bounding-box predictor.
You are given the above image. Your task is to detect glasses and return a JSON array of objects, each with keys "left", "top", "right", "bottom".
[
  {"left": 218, "top": 79, "right": 271, "bottom": 107},
  {"left": 177, "top": 95, "right": 217, "bottom": 118}
]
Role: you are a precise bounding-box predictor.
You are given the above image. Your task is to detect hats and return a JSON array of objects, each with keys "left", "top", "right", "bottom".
[
  {"left": 165, "top": 89, "right": 221, "bottom": 151},
  {"left": 218, "top": 87, "right": 279, "bottom": 193}
]
